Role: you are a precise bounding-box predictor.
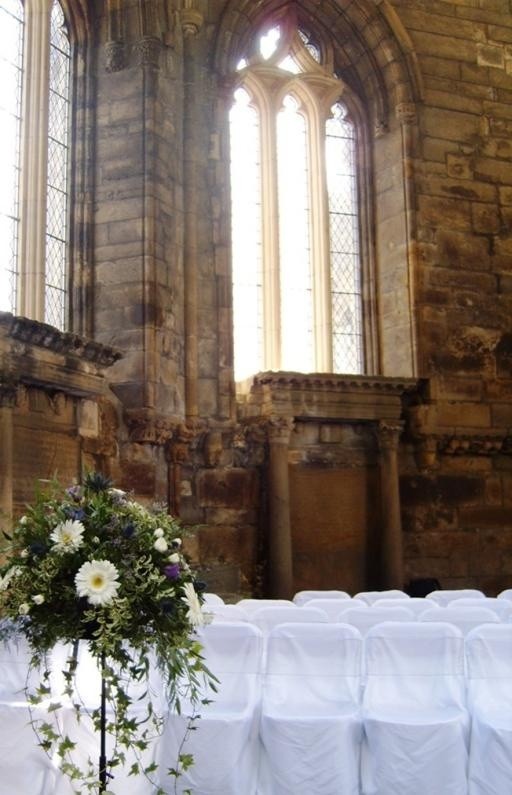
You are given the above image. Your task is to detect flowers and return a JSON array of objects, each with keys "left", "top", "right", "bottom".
[{"left": 0, "top": 468, "right": 212, "bottom": 795}]
